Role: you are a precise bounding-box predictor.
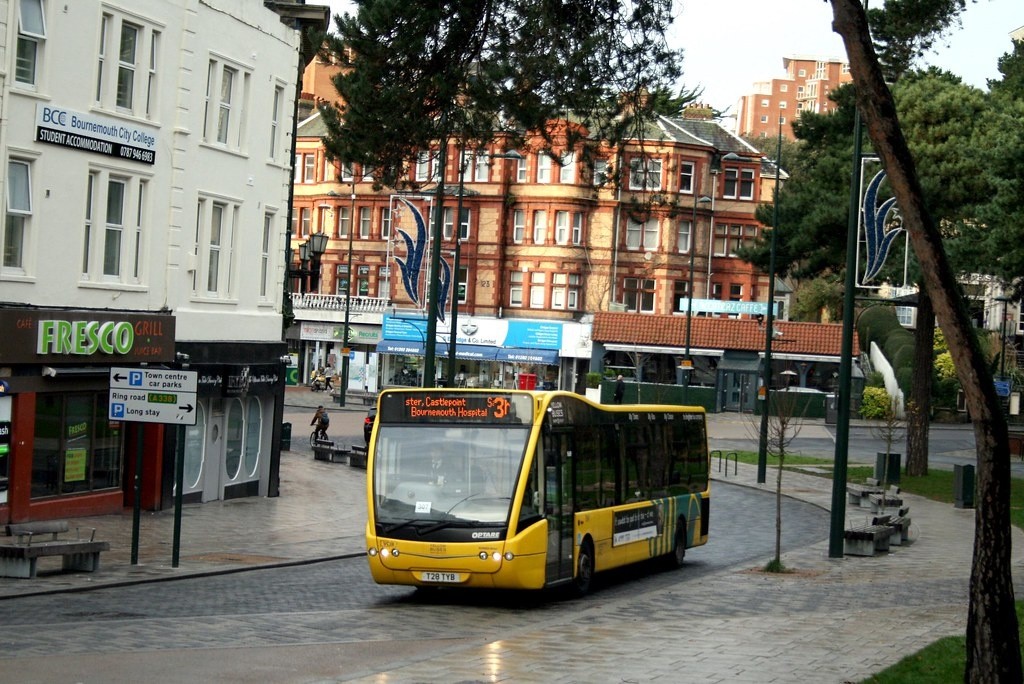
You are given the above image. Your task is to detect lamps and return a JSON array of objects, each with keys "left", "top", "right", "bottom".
[
  {"left": 280, "top": 356, "right": 290, "bottom": 362},
  {"left": 177, "top": 352, "right": 190, "bottom": 360},
  {"left": 601, "top": 357, "right": 611, "bottom": 365}
]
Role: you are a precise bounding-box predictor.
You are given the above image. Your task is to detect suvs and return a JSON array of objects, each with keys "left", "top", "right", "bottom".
[{"left": 364, "top": 406, "right": 377, "bottom": 440}]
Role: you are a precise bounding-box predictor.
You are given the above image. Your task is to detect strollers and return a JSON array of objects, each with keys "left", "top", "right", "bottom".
[{"left": 311, "top": 375, "right": 325, "bottom": 391}]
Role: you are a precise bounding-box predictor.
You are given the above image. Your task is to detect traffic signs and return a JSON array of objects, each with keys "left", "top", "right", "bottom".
[{"left": 107, "top": 367, "right": 198, "bottom": 426}]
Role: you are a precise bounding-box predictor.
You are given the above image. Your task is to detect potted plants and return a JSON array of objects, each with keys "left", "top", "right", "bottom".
[
  {"left": 604, "top": 369, "right": 616, "bottom": 380},
  {"left": 585, "top": 372, "right": 602, "bottom": 404}
]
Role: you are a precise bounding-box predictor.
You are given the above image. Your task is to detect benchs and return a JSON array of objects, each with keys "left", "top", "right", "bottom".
[
  {"left": 311, "top": 439, "right": 350, "bottom": 463},
  {"left": 843, "top": 514, "right": 895, "bottom": 556},
  {"left": 347, "top": 444, "right": 367, "bottom": 469},
  {"left": 869, "top": 484, "right": 904, "bottom": 513},
  {"left": 886, "top": 506, "right": 911, "bottom": 546},
  {"left": 330, "top": 389, "right": 379, "bottom": 406},
  {"left": 847, "top": 478, "right": 881, "bottom": 508},
  {"left": 0, "top": 519, "right": 111, "bottom": 579}
]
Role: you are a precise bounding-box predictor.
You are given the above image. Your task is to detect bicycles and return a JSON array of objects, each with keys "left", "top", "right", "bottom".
[{"left": 309, "top": 423, "right": 331, "bottom": 448}]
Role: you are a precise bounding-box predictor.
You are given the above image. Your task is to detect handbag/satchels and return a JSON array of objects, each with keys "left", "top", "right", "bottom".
[{"left": 320, "top": 413, "right": 329, "bottom": 425}]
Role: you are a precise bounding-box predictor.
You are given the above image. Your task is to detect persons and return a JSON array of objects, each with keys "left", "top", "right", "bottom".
[
  {"left": 310, "top": 363, "right": 335, "bottom": 393},
  {"left": 614, "top": 375, "right": 625, "bottom": 404},
  {"left": 311, "top": 405, "right": 329, "bottom": 445},
  {"left": 543, "top": 373, "right": 556, "bottom": 390}
]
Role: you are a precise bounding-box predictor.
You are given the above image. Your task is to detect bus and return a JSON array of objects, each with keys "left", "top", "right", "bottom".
[{"left": 366, "top": 386, "right": 711, "bottom": 598}]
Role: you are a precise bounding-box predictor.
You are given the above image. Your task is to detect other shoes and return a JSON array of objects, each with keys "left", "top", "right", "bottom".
[
  {"left": 324, "top": 436, "right": 327, "bottom": 439},
  {"left": 330, "top": 389, "right": 334, "bottom": 393},
  {"left": 313, "top": 442, "right": 318, "bottom": 444},
  {"left": 323, "top": 390, "right": 327, "bottom": 392}
]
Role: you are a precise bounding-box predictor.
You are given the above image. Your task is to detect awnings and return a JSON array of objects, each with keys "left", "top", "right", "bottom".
[{"left": 376, "top": 338, "right": 561, "bottom": 366}]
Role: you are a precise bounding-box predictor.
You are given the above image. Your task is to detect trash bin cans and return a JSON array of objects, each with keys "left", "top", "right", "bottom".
[
  {"left": 875, "top": 452, "right": 902, "bottom": 483},
  {"left": 543, "top": 381, "right": 554, "bottom": 390},
  {"left": 519, "top": 373, "right": 536, "bottom": 390},
  {"left": 953, "top": 463, "right": 974, "bottom": 508},
  {"left": 281, "top": 421, "right": 293, "bottom": 451},
  {"left": 825, "top": 394, "right": 839, "bottom": 425}
]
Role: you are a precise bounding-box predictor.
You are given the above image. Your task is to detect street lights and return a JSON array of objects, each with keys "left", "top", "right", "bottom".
[
  {"left": 722, "top": 152, "right": 780, "bottom": 481},
  {"left": 328, "top": 190, "right": 355, "bottom": 406},
  {"left": 992, "top": 295, "right": 1010, "bottom": 423},
  {"left": 449, "top": 148, "right": 521, "bottom": 383},
  {"left": 682, "top": 196, "right": 713, "bottom": 385}
]
